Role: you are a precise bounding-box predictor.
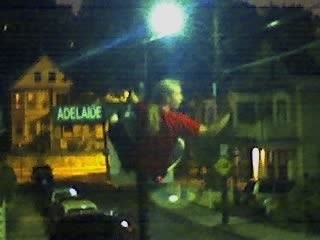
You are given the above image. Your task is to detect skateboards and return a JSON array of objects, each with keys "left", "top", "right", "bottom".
[{"left": 153, "top": 138, "right": 185, "bottom": 182}]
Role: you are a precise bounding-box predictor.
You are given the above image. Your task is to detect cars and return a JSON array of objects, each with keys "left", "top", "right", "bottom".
[
  {"left": 29, "top": 164, "right": 53, "bottom": 189},
  {"left": 52, "top": 199, "right": 96, "bottom": 223},
  {"left": 47, "top": 215, "right": 133, "bottom": 240},
  {"left": 44, "top": 183, "right": 77, "bottom": 208}
]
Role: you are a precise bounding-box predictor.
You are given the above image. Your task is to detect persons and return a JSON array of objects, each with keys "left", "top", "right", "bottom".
[{"left": 107, "top": 78, "right": 232, "bottom": 185}]
